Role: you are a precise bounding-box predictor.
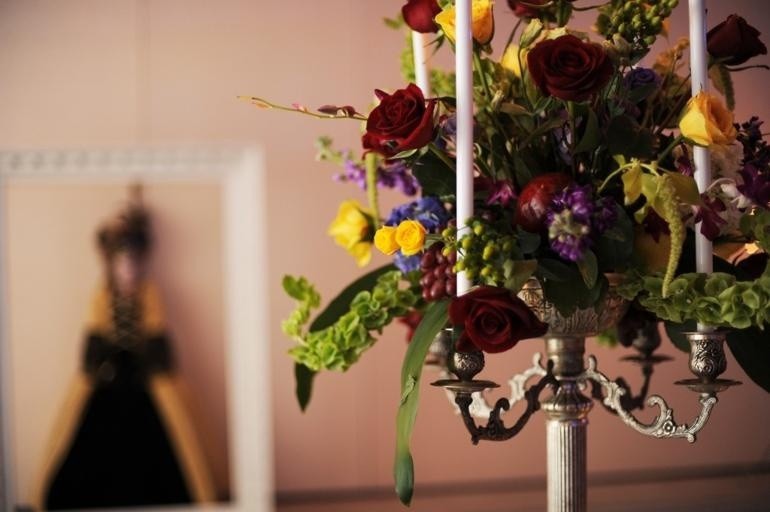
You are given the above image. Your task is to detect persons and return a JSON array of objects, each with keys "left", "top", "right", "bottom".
[{"left": 32, "top": 205, "right": 221, "bottom": 510}]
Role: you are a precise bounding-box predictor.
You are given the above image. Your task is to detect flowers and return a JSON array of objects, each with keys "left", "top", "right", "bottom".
[{"left": 233, "top": 1, "right": 768, "bottom": 507}]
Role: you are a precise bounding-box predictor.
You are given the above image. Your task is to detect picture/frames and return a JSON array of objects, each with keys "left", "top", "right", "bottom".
[{"left": 0, "top": 143, "right": 273, "bottom": 511}]
